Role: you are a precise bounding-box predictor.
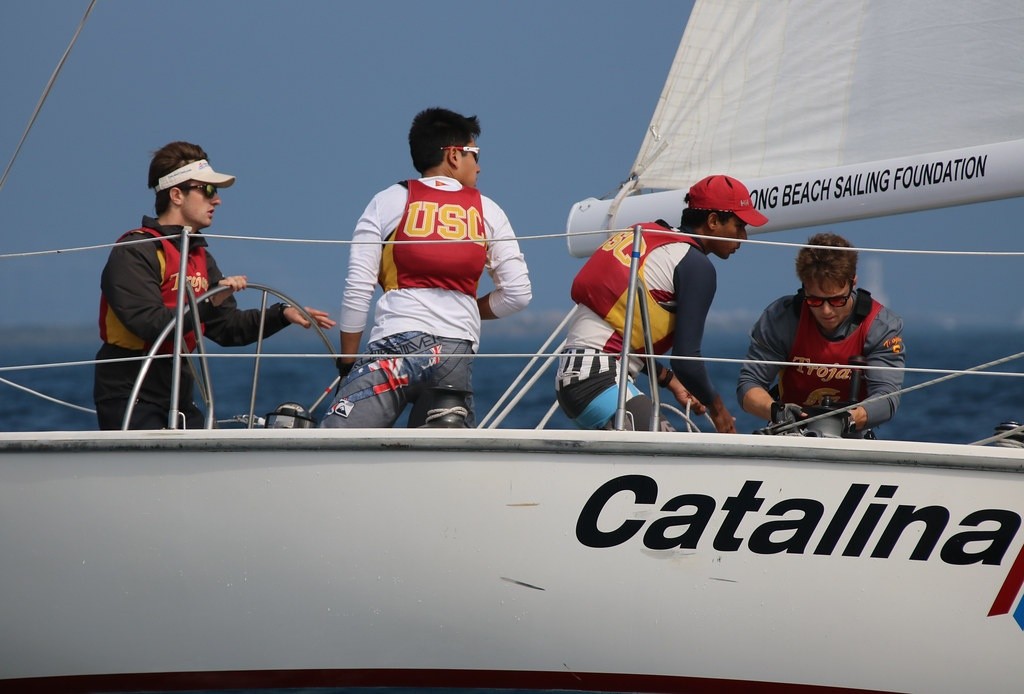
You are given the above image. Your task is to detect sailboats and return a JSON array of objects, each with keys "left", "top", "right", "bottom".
[{"left": 0, "top": 0, "right": 1024, "bottom": 694}]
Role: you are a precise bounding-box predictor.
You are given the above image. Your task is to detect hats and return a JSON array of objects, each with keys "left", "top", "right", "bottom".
[
  {"left": 155, "top": 159, "right": 235, "bottom": 192},
  {"left": 688, "top": 175, "right": 768, "bottom": 227}
]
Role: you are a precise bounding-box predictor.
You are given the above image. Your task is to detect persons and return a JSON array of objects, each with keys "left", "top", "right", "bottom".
[
  {"left": 92, "top": 141, "right": 337, "bottom": 431},
  {"left": 317, "top": 107, "right": 533, "bottom": 428},
  {"left": 556, "top": 175, "right": 769, "bottom": 435},
  {"left": 736, "top": 233, "right": 906, "bottom": 439}
]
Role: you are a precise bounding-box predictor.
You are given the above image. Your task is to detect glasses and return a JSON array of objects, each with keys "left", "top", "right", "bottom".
[
  {"left": 802, "top": 279, "right": 854, "bottom": 307},
  {"left": 177, "top": 183, "right": 218, "bottom": 198},
  {"left": 441, "top": 146, "right": 480, "bottom": 163}
]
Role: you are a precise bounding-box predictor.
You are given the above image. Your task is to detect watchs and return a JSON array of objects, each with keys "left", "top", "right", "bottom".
[{"left": 848, "top": 415, "right": 856, "bottom": 433}]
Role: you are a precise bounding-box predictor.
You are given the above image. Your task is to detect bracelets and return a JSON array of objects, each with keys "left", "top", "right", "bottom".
[{"left": 658, "top": 368, "right": 673, "bottom": 388}]
